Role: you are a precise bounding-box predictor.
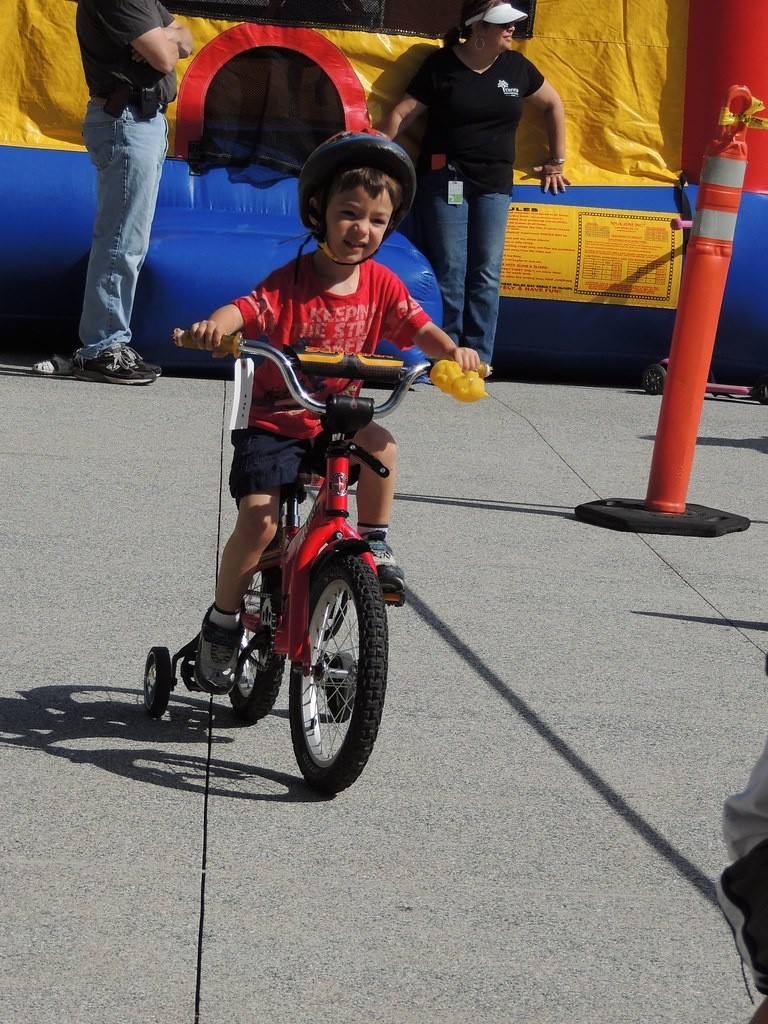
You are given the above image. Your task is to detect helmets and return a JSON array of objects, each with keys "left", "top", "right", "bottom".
[{"left": 298, "top": 130, "right": 417, "bottom": 240}]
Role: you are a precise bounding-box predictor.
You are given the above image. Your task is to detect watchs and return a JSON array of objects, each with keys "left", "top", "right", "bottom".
[{"left": 553, "top": 158, "right": 565, "bottom": 164}]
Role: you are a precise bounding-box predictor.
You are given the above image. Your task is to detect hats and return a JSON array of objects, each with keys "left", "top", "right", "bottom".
[{"left": 465, "top": 3, "right": 528, "bottom": 27}]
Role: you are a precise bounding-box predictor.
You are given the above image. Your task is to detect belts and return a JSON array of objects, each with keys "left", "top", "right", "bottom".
[{"left": 90, "top": 97, "right": 106, "bottom": 106}]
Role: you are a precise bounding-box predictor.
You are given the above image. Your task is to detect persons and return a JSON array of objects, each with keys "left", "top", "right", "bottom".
[
  {"left": 384, "top": 0, "right": 572, "bottom": 376},
  {"left": 76, "top": 0, "right": 195, "bottom": 387},
  {"left": 191, "top": 154, "right": 482, "bottom": 686},
  {"left": 719, "top": 649, "right": 767, "bottom": 1024}
]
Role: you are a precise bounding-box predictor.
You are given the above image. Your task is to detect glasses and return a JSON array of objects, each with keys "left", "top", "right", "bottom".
[{"left": 498, "top": 22, "right": 515, "bottom": 30}]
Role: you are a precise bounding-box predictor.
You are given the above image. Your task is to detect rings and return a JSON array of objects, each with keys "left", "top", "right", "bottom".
[{"left": 557, "top": 177, "right": 562, "bottom": 178}]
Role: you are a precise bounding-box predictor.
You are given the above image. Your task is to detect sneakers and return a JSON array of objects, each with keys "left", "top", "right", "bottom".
[
  {"left": 121, "top": 346, "right": 162, "bottom": 376},
  {"left": 33, "top": 354, "right": 76, "bottom": 375},
  {"left": 193, "top": 604, "right": 246, "bottom": 695},
  {"left": 76, "top": 348, "right": 156, "bottom": 384},
  {"left": 361, "top": 530, "right": 404, "bottom": 595}
]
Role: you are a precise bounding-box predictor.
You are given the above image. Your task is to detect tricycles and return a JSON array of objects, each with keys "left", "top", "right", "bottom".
[{"left": 143, "top": 325, "right": 493, "bottom": 794}]
